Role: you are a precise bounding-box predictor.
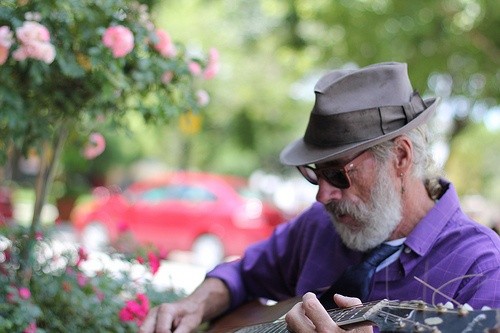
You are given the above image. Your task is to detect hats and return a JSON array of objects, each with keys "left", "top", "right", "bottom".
[{"left": 280, "top": 61, "right": 441, "bottom": 167}]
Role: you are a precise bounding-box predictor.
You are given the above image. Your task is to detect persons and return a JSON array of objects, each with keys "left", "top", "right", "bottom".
[{"left": 139, "top": 62, "right": 499, "bottom": 333}]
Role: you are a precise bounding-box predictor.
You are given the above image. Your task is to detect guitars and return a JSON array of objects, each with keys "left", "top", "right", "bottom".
[{"left": 204, "top": 274, "right": 500, "bottom": 333}]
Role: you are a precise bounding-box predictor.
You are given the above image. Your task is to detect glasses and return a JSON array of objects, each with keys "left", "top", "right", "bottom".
[{"left": 296, "top": 148, "right": 375, "bottom": 189}]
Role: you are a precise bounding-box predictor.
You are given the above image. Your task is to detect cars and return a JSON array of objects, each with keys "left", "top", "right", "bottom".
[{"left": 68, "top": 170, "right": 298, "bottom": 262}]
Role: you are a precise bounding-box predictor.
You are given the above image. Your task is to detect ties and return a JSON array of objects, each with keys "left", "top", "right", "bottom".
[{"left": 318, "top": 242, "right": 403, "bottom": 309}]
